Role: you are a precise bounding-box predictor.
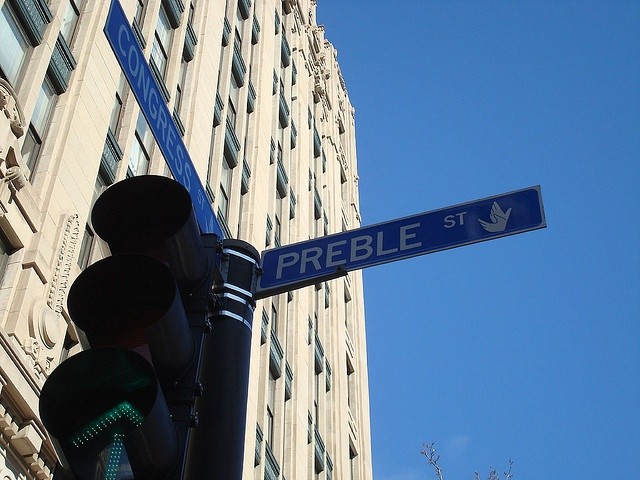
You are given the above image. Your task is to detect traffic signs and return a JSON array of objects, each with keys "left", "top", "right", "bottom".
[
  {"left": 256, "top": 186, "right": 546, "bottom": 299},
  {"left": 101, "top": 0, "right": 225, "bottom": 242}
]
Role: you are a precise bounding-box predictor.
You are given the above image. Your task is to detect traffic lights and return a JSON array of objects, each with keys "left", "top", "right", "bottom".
[{"left": 38, "top": 175, "right": 219, "bottom": 480}]
